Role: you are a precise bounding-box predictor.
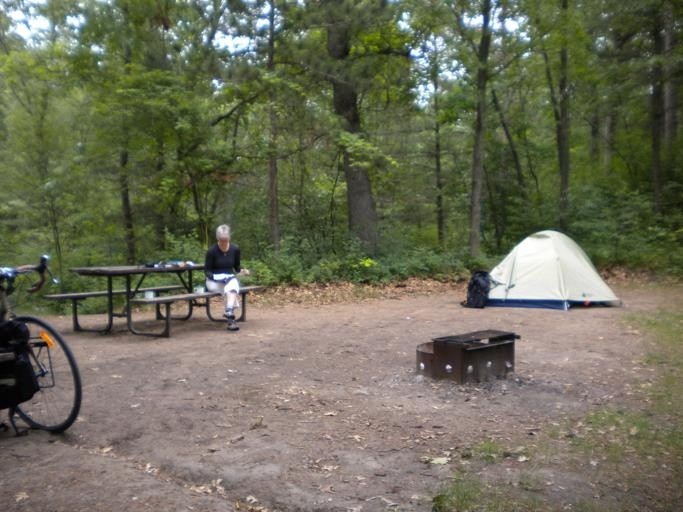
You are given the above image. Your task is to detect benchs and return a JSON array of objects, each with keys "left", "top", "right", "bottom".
[
  {"left": 130, "top": 286, "right": 263, "bottom": 337},
  {"left": 43, "top": 284, "right": 193, "bottom": 333}
]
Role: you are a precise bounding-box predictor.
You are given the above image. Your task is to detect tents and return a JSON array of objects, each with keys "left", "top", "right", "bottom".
[{"left": 460, "top": 229, "right": 622, "bottom": 311}]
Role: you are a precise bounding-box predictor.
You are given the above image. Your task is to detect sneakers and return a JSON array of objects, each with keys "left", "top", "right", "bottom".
[
  {"left": 224, "top": 308, "right": 234, "bottom": 319},
  {"left": 227, "top": 322, "right": 239, "bottom": 330}
]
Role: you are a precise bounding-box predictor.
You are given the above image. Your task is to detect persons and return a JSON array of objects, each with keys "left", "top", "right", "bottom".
[{"left": 203, "top": 223, "right": 241, "bottom": 331}]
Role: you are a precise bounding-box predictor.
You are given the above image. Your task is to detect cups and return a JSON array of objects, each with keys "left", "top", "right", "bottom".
[
  {"left": 193, "top": 287, "right": 203, "bottom": 294},
  {"left": 144, "top": 292, "right": 152, "bottom": 300}
]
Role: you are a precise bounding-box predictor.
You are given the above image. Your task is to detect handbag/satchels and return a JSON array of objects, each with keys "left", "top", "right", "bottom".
[{"left": 1, "top": 322, "right": 40, "bottom": 410}]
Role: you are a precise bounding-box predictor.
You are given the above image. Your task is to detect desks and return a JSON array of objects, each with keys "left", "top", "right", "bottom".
[{"left": 68, "top": 264, "right": 209, "bottom": 337}]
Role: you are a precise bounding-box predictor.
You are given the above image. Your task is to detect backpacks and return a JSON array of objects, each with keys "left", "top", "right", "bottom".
[{"left": 467, "top": 270, "right": 489, "bottom": 308}]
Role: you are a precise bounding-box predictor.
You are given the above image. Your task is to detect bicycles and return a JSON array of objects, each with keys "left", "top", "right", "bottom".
[{"left": 0, "top": 255, "right": 81, "bottom": 439}]
